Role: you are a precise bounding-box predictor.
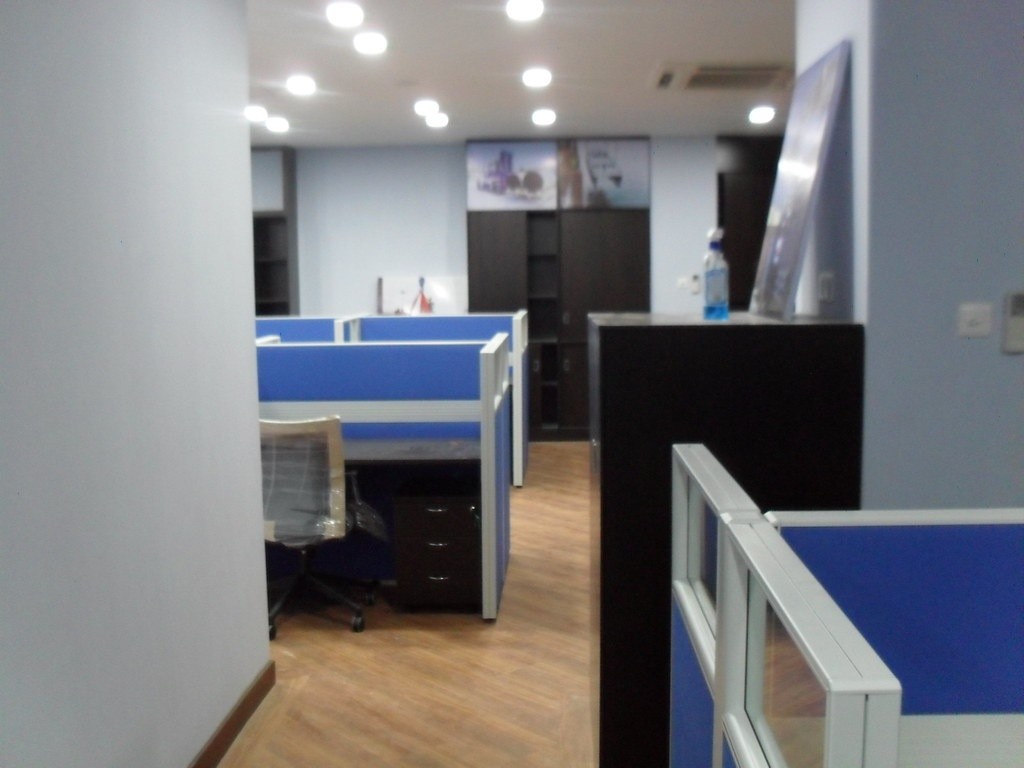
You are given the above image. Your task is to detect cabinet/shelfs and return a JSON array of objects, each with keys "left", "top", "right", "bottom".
[
  {"left": 468, "top": 208, "right": 650, "bottom": 443},
  {"left": 252, "top": 142, "right": 300, "bottom": 316},
  {"left": 588, "top": 311, "right": 867, "bottom": 768}
]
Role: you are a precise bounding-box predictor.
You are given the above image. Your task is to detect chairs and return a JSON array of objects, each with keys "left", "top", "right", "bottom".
[{"left": 259, "top": 415, "right": 390, "bottom": 639}]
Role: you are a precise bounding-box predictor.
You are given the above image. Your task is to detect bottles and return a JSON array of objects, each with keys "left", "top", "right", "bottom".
[{"left": 700, "top": 241, "right": 729, "bottom": 320}]
